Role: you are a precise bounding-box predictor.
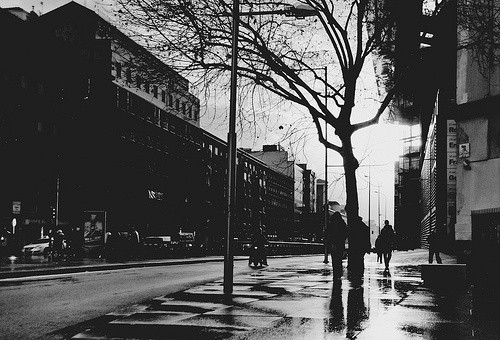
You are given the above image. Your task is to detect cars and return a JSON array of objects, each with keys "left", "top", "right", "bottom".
[{"left": 21, "top": 238, "right": 55, "bottom": 258}]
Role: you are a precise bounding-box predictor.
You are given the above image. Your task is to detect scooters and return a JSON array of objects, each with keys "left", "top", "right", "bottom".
[{"left": 252, "top": 244, "right": 269, "bottom": 269}]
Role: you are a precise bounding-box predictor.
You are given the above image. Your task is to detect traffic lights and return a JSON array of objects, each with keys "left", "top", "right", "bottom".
[{"left": 49, "top": 208, "right": 56, "bottom": 220}]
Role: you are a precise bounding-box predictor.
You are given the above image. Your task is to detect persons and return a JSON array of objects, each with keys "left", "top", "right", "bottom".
[
  {"left": 324, "top": 212, "right": 370, "bottom": 276},
  {"left": 131, "top": 227, "right": 140, "bottom": 261},
  {"left": 428, "top": 228, "right": 442, "bottom": 264},
  {"left": 249, "top": 235, "right": 270, "bottom": 265},
  {"left": 381, "top": 220, "right": 394, "bottom": 269},
  {"left": 54, "top": 229, "right": 64, "bottom": 263},
  {"left": 375, "top": 235, "right": 382, "bottom": 263}
]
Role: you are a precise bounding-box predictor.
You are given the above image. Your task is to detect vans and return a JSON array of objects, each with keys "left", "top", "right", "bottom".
[{"left": 143, "top": 235, "right": 171, "bottom": 248}]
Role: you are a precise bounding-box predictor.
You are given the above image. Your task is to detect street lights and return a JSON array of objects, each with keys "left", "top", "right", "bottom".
[{"left": 222, "top": 0, "right": 318, "bottom": 295}]
